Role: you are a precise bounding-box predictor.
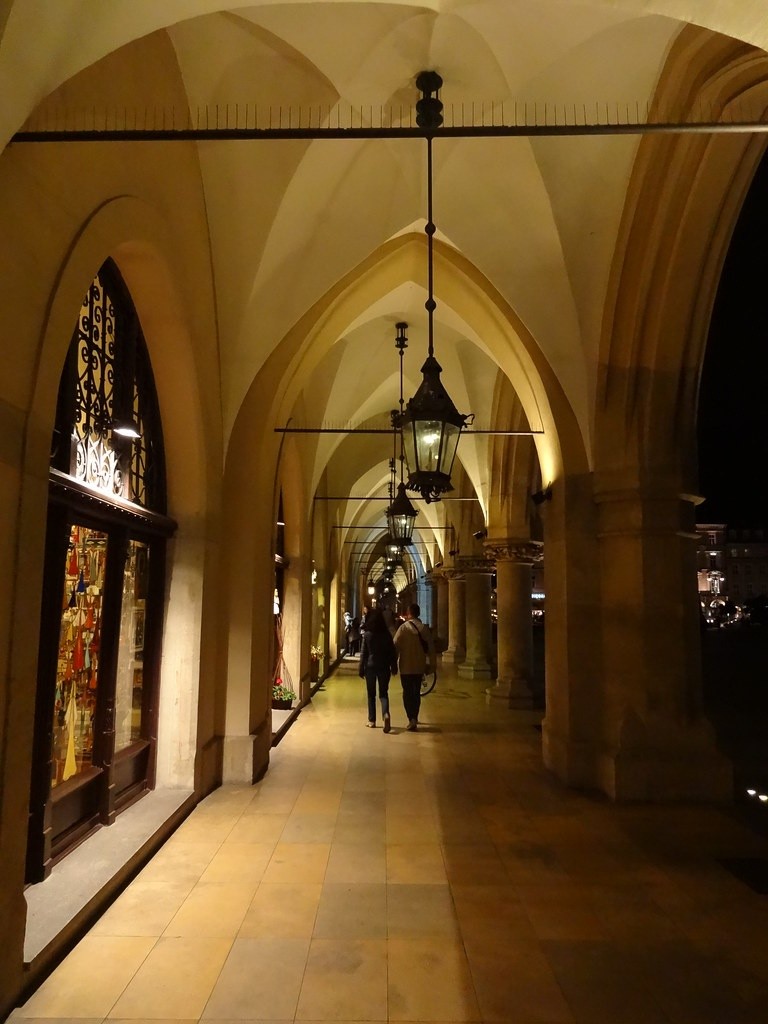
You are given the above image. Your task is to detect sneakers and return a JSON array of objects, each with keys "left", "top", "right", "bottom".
[
  {"left": 365, "top": 720, "right": 376, "bottom": 728},
  {"left": 407, "top": 719, "right": 417, "bottom": 729},
  {"left": 383, "top": 713, "right": 391, "bottom": 733}
]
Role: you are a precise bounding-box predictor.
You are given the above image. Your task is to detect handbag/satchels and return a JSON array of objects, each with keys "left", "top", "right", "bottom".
[{"left": 410, "top": 621, "right": 428, "bottom": 653}]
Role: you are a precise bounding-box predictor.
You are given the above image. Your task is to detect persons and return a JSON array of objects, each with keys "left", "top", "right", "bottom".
[
  {"left": 343, "top": 611, "right": 357, "bottom": 655},
  {"left": 393, "top": 604, "right": 438, "bottom": 730},
  {"left": 360, "top": 608, "right": 370, "bottom": 647},
  {"left": 355, "top": 613, "right": 398, "bottom": 734}
]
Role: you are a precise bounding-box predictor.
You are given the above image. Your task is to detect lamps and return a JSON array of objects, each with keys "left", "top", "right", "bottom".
[
  {"left": 385, "top": 416, "right": 418, "bottom": 547},
  {"left": 368, "top": 573, "right": 375, "bottom": 595},
  {"left": 392, "top": 70, "right": 477, "bottom": 500},
  {"left": 381, "top": 480, "right": 404, "bottom": 597}
]
